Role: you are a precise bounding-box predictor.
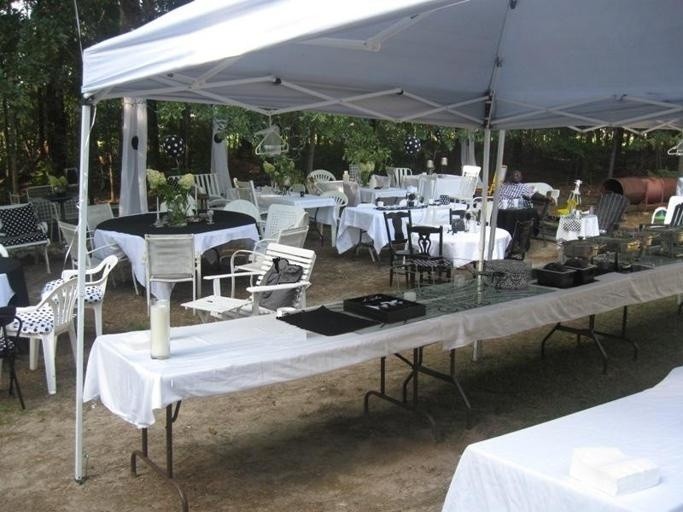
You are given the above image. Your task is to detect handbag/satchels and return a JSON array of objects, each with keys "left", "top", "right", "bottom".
[{"left": 256, "top": 258, "right": 301, "bottom": 310}]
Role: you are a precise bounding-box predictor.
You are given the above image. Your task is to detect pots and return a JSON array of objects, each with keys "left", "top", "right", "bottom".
[
  {"left": 615, "top": 230, "right": 654, "bottom": 246},
  {"left": 560, "top": 235, "right": 607, "bottom": 264},
  {"left": 655, "top": 228, "right": 683, "bottom": 244},
  {"left": 588, "top": 234, "right": 640, "bottom": 255}
]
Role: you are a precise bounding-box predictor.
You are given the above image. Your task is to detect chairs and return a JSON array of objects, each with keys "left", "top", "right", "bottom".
[
  {"left": 393, "top": 168, "right": 413, "bottom": 188},
  {"left": 407, "top": 224, "right": 453, "bottom": 289},
  {"left": 41, "top": 255, "right": 120, "bottom": 338},
  {"left": 168, "top": 173, "right": 230, "bottom": 209},
  {"left": 650, "top": 196, "right": 683, "bottom": 224},
  {"left": 305, "top": 170, "right": 336, "bottom": 194},
  {"left": 383, "top": 211, "right": 412, "bottom": 287},
  {"left": 449, "top": 208, "right": 482, "bottom": 225},
  {"left": 6, "top": 277, "right": 78, "bottom": 394},
  {"left": 317, "top": 181, "right": 361, "bottom": 207},
  {"left": 58, "top": 222, "right": 140, "bottom": 297},
  {"left": 204, "top": 242, "right": 318, "bottom": 320},
  {"left": 258, "top": 194, "right": 337, "bottom": 247},
  {"left": 0, "top": 293, "right": 27, "bottom": 411},
  {"left": 505, "top": 218, "right": 536, "bottom": 260},
  {"left": 593, "top": 192, "right": 630, "bottom": 236},
  {"left": 669, "top": 202, "right": 683, "bottom": 226},
  {"left": 0, "top": 244, "right": 8, "bottom": 257},
  {"left": 235, "top": 203, "right": 310, "bottom": 287},
  {"left": 234, "top": 177, "right": 260, "bottom": 211},
  {"left": 143, "top": 232, "right": 199, "bottom": 315},
  {"left": 462, "top": 165, "right": 482, "bottom": 195},
  {"left": 30, "top": 198, "right": 60, "bottom": 242},
  {"left": 82, "top": 203, "right": 114, "bottom": 257},
  {"left": 60, "top": 197, "right": 84, "bottom": 220},
  {"left": 0, "top": 202, "right": 52, "bottom": 273}
]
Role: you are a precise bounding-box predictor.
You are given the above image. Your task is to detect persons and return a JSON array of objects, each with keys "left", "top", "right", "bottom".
[{"left": 493, "top": 170, "right": 555, "bottom": 259}]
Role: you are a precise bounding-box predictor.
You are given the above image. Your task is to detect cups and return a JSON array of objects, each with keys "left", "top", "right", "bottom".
[
  {"left": 149, "top": 297, "right": 173, "bottom": 361},
  {"left": 377, "top": 202, "right": 383, "bottom": 206}
]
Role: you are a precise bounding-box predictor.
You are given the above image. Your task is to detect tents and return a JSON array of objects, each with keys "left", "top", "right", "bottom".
[{"left": 78, "top": 0, "right": 683, "bottom": 484}]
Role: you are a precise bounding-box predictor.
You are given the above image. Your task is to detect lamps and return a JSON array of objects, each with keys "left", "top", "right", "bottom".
[
  {"left": 427, "top": 160, "right": 434, "bottom": 175},
  {"left": 440, "top": 158, "right": 448, "bottom": 174}
]
